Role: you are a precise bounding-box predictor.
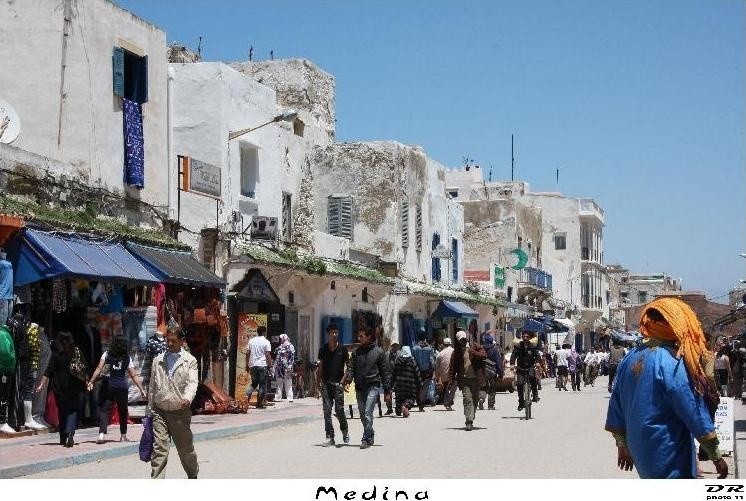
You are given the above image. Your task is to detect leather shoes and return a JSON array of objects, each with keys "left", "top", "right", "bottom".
[{"left": 321, "top": 398, "right": 526, "bottom": 449}]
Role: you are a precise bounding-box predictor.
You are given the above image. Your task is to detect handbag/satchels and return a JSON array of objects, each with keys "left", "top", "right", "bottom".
[
  {"left": 193, "top": 309, "right": 219, "bottom": 325},
  {"left": 427, "top": 380, "right": 435, "bottom": 399},
  {"left": 98, "top": 364, "right": 111, "bottom": 379},
  {"left": 168, "top": 317, "right": 179, "bottom": 328},
  {"left": 70, "top": 358, "right": 86, "bottom": 384},
  {"left": 139, "top": 417, "right": 153, "bottom": 462},
  {"left": 482, "top": 359, "right": 497, "bottom": 379}
]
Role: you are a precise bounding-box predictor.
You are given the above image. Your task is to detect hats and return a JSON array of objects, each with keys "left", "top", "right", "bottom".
[
  {"left": 485, "top": 336, "right": 493, "bottom": 343},
  {"left": 456, "top": 331, "right": 466, "bottom": 340}
]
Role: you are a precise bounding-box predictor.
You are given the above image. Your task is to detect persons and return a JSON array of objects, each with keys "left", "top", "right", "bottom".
[
  {"left": 146, "top": 328, "right": 199, "bottom": 479},
  {"left": 604, "top": 298, "right": 728, "bottom": 479},
  {"left": 0, "top": 325, "right": 17, "bottom": 434},
  {"left": 713, "top": 335, "right": 746, "bottom": 399},
  {"left": 35, "top": 330, "right": 92, "bottom": 447},
  {"left": 0, "top": 252, "right": 14, "bottom": 324},
  {"left": 246, "top": 326, "right": 272, "bottom": 409},
  {"left": 316, "top": 326, "right": 635, "bottom": 448},
  {"left": 697, "top": 333, "right": 721, "bottom": 461},
  {"left": 20, "top": 322, "right": 47, "bottom": 430},
  {"left": 88, "top": 334, "right": 146, "bottom": 444},
  {"left": 33, "top": 326, "right": 51, "bottom": 427},
  {"left": 272, "top": 334, "right": 295, "bottom": 402},
  {"left": 146, "top": 331, "right": 168, "bottom": 358}
]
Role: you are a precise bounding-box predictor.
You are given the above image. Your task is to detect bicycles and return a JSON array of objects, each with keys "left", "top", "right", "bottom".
[{"left": 510, "top": 365, "right": 540, "bottom": 420}]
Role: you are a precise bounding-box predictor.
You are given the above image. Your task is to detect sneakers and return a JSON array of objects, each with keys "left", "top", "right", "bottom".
[{"left": 60, "top": 431, "right": 128, "bottom": 447}]
